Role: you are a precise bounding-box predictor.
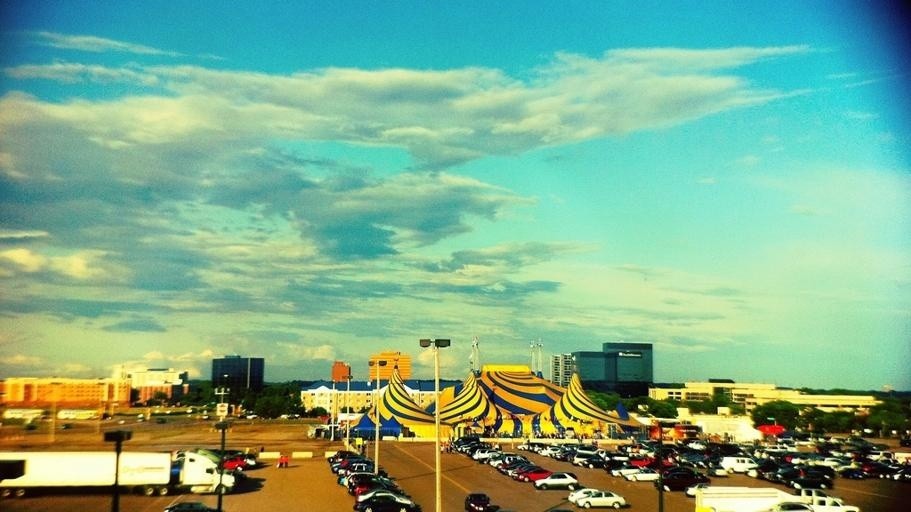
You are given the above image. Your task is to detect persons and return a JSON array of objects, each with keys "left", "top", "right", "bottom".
[
  {"left": 440, "top": 438, "right": 454, "bottom": 456},
  {"left": 285, "top": 454, "right": 290, "bottom": 468},
  {"left": 261, "top": 445, "right": 265, "bottom": 452},
  {"left": 278, "top": 455, "right": 285, "bottom": 467},
  {"left": 254, "top": 448, "right": 258, "bottom": 455}
]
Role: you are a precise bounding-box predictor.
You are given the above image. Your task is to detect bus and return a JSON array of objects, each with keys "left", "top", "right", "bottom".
[{"left": 2, "top": 407, "right": 47, "bottom": 423}]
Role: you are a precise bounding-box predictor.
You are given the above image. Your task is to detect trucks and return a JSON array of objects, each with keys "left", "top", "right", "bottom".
[
  {"left": 0, "top": 450, "right": 239, "bottom": 498},
  {"left": 693, "top": 487, "right": 860, "bottom": 512}
]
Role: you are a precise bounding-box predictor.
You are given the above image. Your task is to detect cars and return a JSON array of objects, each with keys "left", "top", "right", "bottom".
[
  {"left": 171, "top": 443, "right": 258, "bottom": 472},
  {"left": 328, "top": 447, "right": 424, "bottom": 512},
  {"left": 42, "top": 400, "right": 303, "bottom": 432},
  {"left": 457, "top": 426, "right": 910, "bottom": 492},
  {"left": 575, "top": 492, "right": 628, "bottom": 512},
  {"left": 162, "top": 499, "right": 225, "bottom": 512},
  {"left": 567, "top": 488, "right": 601, "bottom": 504},
  {"left": 463, "top": 493, "right": 492, "bottom": 511}
]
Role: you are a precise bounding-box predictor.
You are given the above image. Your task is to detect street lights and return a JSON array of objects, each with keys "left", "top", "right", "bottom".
[
  {"left": 97, "top": 426, "right": 135, "bottom": 512},
  {"left": 340, "top": 374, "right": 353, "bottom": 450},
  {"left": 766, "top": 416, "right": 777, "bottom": 427},
  {"left": 417, "top": 337, "right": 453, "bottom": 512},
  {"left": 365, "top": 359, "right": 388, "bottom": 476},
  {"left": 212, "top": 418, "right": 231, "bottom": 512}
]
